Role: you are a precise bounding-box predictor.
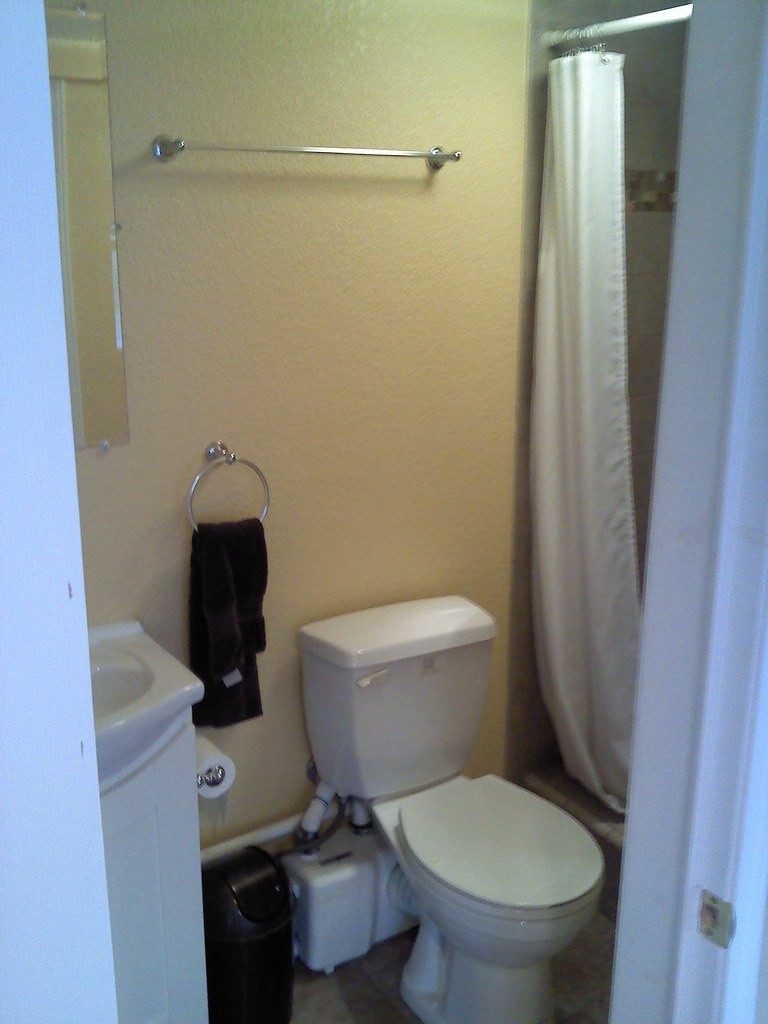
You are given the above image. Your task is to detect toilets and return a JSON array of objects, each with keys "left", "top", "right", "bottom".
[{"left": 294, "top": 592, "right": 608, "bottom": 1024}]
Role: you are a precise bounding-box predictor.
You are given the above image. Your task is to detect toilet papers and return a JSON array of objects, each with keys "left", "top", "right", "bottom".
[{"left": 193, "top": 734, "right": 237, "bottom": 829}]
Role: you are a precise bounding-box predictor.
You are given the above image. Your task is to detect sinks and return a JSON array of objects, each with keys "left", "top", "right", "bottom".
[{"left": 88, "top": 641, "right": 155, "bottom": 721}]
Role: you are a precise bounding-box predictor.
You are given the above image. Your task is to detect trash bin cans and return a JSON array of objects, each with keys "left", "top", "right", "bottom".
[{"left": 201, "top": 845, "right": 294, "bottom": 1024}]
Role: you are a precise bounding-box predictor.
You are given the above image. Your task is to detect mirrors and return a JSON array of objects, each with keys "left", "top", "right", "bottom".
[{"left": 44, "top": 8, "right": 130, "bottom": 448}]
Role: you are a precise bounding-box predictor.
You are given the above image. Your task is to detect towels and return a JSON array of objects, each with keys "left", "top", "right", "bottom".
[{"left": 187, "top": 517, "right": 268, "bottom": 730}]
